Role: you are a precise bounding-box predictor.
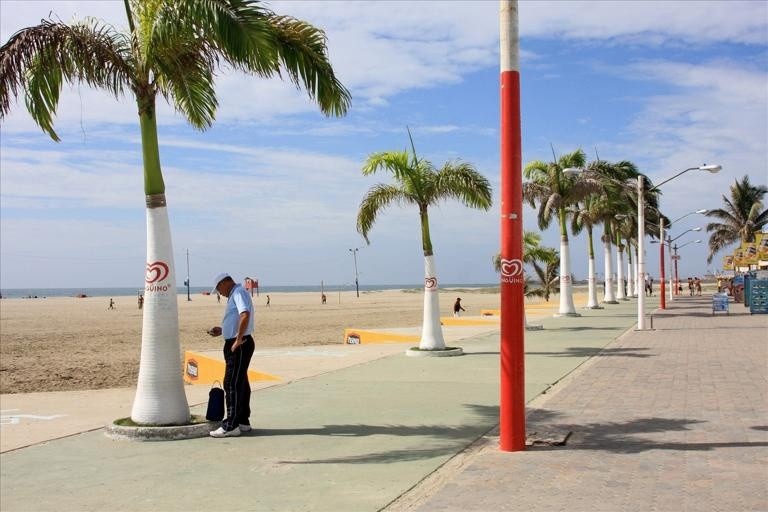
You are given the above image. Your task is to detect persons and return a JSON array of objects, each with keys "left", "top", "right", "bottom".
[
  {"left": 645, "top": 273, "right": 654, "bottom": 297},
  {"left": 718, "top": 279, "right": 721, "bottom": 292},
  {"left": 217, "top": 293, "right": 220, "bottom": 303},
  {"left": 265, "top": 295, "right": 270, "bottom": 308},
  {"left": 138, "top": 295, "right": 144, "bottom": 309},
  {"left": 688, "top": 277, "right": 701, "bottom": 296},
  {"left": 322, "top": 295, "right": 326, "bottom": 304},
  {"left": 453, "top": 298, "right": 465, "bottom": 317},
  {"left": 678, "top": 280, "right": 682, "bottom": 295},
  {"left": 210, "top": 271, "right": 254, "bottom": 438},
  {"left": 108, "top": 299, "right": 113, "bottom": 309}
]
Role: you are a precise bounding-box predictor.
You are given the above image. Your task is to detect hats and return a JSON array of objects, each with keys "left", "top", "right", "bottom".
[{"left": 210, "top": 272, "right": 231, "bottom": 296}]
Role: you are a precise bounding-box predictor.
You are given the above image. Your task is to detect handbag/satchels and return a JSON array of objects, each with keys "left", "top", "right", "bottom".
[{"left": 205, "top": 380, "right": 225, "bottom": 421}]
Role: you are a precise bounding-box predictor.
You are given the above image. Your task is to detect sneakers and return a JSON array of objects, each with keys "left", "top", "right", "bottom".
[{"left": 209, "top": 421, "right": 252, "bottom": 438}]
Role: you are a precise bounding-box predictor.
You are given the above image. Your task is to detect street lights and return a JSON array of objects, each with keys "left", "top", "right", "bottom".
[
  {"left": 562, "top": 164, "right": 722, "bottom": 331},
  {"left": 349, "top": 248, "right": 359, "bottom": 297}
]
